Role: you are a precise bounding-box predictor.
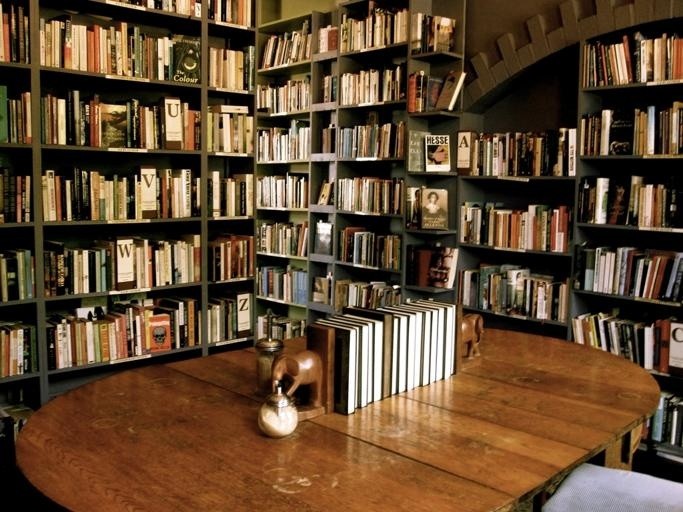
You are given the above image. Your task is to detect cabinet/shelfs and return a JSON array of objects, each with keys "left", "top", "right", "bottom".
[
  {"left": 0, "top": 0, "right": 256, "bottom": 407},
  {"left": 573, "top": 39, "right": 683, "bottom": 466},
  {"left": 457, "top": 113, "right": 573, "bottom": 340},
  {"left": 256, "top": 0, "right": 457, "bottom": 342}
]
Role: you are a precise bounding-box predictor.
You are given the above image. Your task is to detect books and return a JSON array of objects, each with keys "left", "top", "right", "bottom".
[
  {"left": 313, "top": 300, "right": 456, "bottom": 416},
  {"left": 459, "top": 127, "right": 577, "bottom": 323},
  {"left": 0, "top": 0, "right": 255, "bottom": 377},
  {"left": 571, "top": 31, "right": 682, "bottom": 445},
  {"left": 254, "top": 9, "right": 466, "bottom": 341}
]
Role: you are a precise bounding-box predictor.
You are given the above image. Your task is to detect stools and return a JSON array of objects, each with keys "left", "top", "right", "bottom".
[{"left": 532, "top": 461, "right": 683, "bottom": 512}]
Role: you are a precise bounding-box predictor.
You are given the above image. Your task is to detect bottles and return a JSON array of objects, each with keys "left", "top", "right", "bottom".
[
  {"left": 254, "top": 384, "right": 299, "bottom": 439},
  {"left": 252, "top": 313, "right": 284, "bottom": 390}
]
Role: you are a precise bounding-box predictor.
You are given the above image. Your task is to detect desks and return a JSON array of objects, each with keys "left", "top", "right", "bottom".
[{"left": 14, "top": 314, "right": 661, "bottom": 512}]
